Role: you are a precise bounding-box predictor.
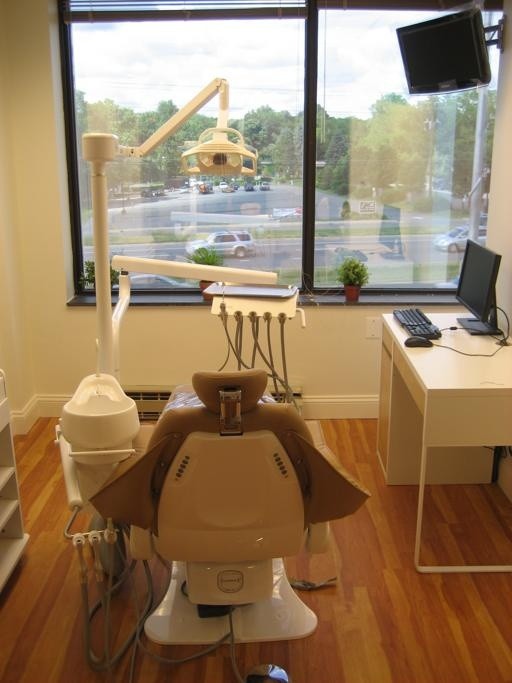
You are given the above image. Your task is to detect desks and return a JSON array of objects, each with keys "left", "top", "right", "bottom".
[
  {"left": 376, "top": 312, "right": 512, "bottom": 573},
  {"left": 325, "top": 242, "right": 414, "bottom": 284}
]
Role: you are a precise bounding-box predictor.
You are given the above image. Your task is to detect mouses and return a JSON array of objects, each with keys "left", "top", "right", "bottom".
[{"left": 404, "top": 337, "right": 432, "bottom": 347}]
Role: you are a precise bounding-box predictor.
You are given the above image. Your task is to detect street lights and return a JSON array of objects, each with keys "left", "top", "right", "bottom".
[{"left": 422, "top": 117, "right": 444, "bottom": 195}]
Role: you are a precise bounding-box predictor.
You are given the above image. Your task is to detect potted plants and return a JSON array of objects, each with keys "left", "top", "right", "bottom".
[
  {"left": 335, "top": 256, "right": 370, "bottom": 301},
  {"left": 182, "top": 242, "right": 223, "bottom": 300},
  {"left": 76, "top": 260, "right": 119, "bottom": 295}
]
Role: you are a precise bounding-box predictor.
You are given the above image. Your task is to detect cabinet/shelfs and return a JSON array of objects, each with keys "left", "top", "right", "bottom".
[{"left": 0, "top": 367, "right": 30, "bottom": 598}]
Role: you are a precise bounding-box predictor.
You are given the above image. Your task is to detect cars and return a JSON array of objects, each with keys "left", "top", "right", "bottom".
[
  {"left": 479, "top": 212, "right": 488, "bottom": 224},
  {"left": 185, "top": 177, "right": 273, "bottom": 192},
  {"left": 139, "top": 189, "right": 160, "bottom": 197},
  {"left": 111, "top": 272, "right": 195, "bottom": 288}
]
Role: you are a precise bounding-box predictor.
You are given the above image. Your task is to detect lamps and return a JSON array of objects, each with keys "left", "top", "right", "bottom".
[{"left": 118, "top": 76, "right": 258, "bottom": 177}]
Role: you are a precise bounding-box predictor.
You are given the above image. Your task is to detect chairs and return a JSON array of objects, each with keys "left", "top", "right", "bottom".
[{"left": 88, "top": 368, "right": 373, "bottom": 644}]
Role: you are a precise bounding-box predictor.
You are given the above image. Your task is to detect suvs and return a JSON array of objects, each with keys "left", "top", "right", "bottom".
[{"left": 183, "top": 229, "right": 258, "bottom": 260}]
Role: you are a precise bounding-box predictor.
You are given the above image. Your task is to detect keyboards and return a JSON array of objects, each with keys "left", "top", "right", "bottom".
[{"left": 393, "top": 308, "right": 441, "bottom": 340}]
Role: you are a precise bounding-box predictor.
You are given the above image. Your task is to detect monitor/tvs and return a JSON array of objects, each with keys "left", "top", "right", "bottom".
[
  {"left": 456, "top": 239, "right": 502, "bottom": 335},
  {"left": 396, "top": 9, "right": 490, "bottom": 95}
]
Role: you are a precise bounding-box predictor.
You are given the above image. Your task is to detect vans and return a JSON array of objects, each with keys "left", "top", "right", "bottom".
[{"left": 430, "top": 224, "right": 487, "bottom": 252}]
